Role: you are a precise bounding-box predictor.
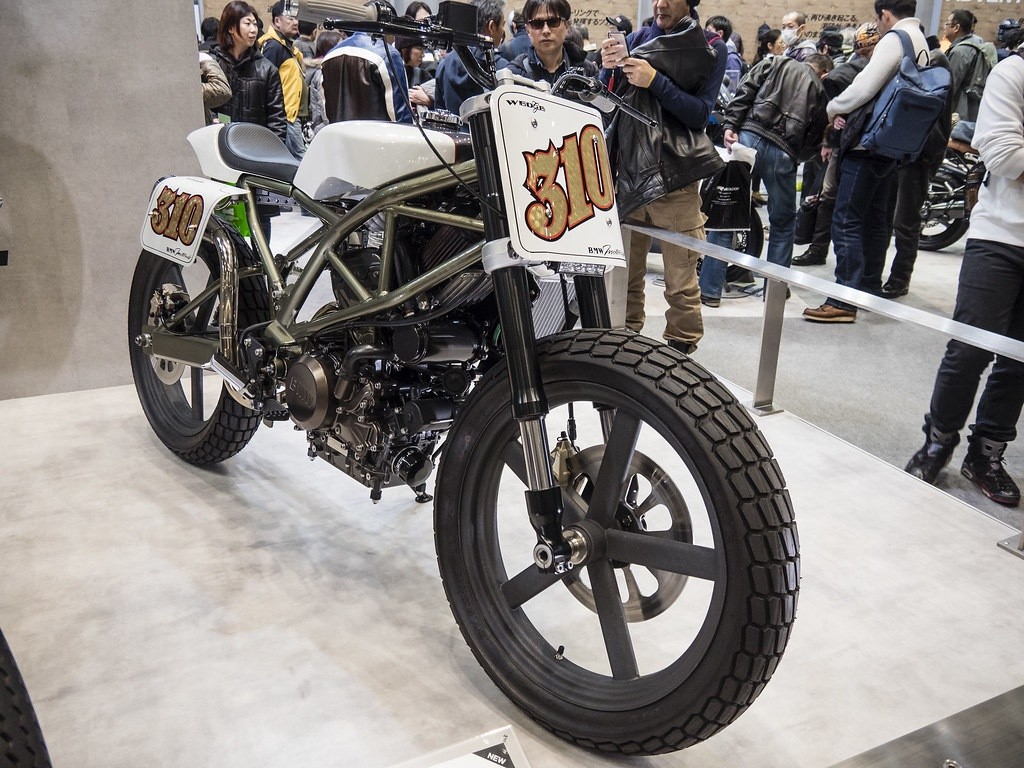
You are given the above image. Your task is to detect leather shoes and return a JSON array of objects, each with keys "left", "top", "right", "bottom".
[
  {"left": 881, "top": 281, "right": 909, "bottom": 298},
  {"left": 792, "top": 250, "right": 827, "bottom": 266}
]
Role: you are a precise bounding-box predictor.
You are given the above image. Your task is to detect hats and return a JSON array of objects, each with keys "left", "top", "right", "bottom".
[
  {"left": 815, "top": 30, "right": 844, "bottom": 49},
  {"left": 606, "top": 14, "right": 633, "bottom": 36}
]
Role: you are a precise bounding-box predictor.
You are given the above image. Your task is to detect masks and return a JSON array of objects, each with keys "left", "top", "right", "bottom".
[{"left": 782, "top": 24, "right": 806, "bottom": 46}]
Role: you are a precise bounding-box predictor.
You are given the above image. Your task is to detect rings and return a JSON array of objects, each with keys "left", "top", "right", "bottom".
[{"left": 628, "top": 73, "right": 632, "bottom": 79}]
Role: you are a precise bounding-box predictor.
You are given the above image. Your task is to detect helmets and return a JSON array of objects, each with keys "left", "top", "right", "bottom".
[{"left": 998, "top": 19, "right": 1021, "bottom": 41}]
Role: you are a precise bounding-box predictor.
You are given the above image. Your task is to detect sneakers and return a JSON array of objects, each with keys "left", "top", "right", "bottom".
[
  {"left": 902, "top": 413, "right": 960, "bottom": 485},
  {"left": 961, "top": 434, "right": 1021, "bottom": 508},
  {"left": 802, "top": 304, "right": 856, "bottom": 323}
]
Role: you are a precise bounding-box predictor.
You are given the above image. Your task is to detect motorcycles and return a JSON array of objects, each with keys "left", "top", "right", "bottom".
[
  {"left": 917, "top": 145, "right": 984, "bottom": 251},
  {"left": 129, "top": 0, "right": 801, "bottom": 755}
]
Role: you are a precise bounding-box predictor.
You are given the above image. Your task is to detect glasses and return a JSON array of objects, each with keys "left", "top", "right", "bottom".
[{"left": 525, "top": 17, "right": 563, "bottom": 30}]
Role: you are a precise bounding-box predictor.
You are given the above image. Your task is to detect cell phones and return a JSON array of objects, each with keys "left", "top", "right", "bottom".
[{"left": 608, "top": 30, "right": 631, "bottom": 67}]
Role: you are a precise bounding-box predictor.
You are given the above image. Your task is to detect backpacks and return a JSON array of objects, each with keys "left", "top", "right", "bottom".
[
  {"left": 860, "top": 29, "right": 952, "bottom": 164},
  {"left": 955, "top": 40, "right": 998, "bottom": 101},
  {"left": 729, "top": 51, "right": 753, "bottom": 76}
]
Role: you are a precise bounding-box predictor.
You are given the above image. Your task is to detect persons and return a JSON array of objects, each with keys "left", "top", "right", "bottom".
[
  {"left": 195, "top": 0, "right": 1024, "bottom": 354},
  {"left": 897, "top": 40, "right": 1024, "bottom": 507}
]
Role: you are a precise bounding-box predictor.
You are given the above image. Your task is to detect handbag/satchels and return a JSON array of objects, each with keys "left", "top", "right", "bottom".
[{"left": 700, "top": 143, "right": 757, "bottom": 232}]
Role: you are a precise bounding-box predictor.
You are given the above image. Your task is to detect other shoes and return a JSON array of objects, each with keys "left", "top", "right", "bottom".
[{"left": 700, "top": 294, "right": 720, "bottom": 308}]
